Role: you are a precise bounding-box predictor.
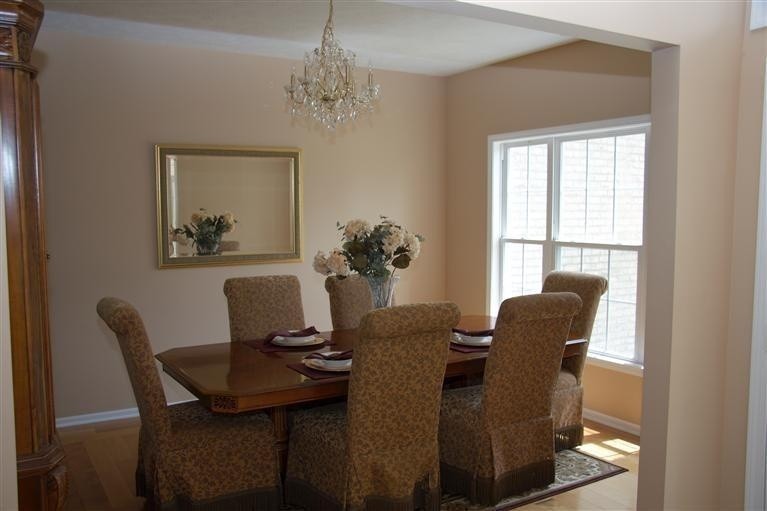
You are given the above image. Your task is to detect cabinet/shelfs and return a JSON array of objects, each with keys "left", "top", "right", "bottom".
[{"left": 0, "top": 0, "right": 78, "bottom": 511}]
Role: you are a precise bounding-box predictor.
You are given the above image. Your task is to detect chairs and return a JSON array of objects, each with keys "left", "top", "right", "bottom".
[
  {"left": 325, "top": 270, "right": 397, "bottom": 330},
  {"left": 541, "top": 269, "right": 609, "bottom": 452},
  {"left": 223, "top": 275, "right": 305, "bottom": 343},
  {"left": 284, "top": 303, "right": 461, "bottom": 511},
  {"left": 96, "top": 297, "right": 284, "bottom": 511},
  {"left": 438, "top": 292, "right": 583, "bottom": 507}
]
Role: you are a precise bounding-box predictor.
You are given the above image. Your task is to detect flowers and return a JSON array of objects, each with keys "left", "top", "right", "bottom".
[
  {"left": 312, "top": 217, "right": 425, "bottom": 307},
  {"left": 168, "top": 208, "right": 240, "bottom": 254}
]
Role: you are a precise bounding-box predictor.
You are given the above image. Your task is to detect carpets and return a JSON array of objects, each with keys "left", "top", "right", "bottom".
[{"left": 440, "top": 448, "right": 629, "bottom": 511}]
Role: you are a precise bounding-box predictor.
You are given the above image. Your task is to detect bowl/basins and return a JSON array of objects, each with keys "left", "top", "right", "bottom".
[
  {"left": 283, "top": 330, "right": 316, "bottom": 343},
  {"left": 460, "top": 335, "right": 492, "bottom": 344},
  {"left": 316, "top": 351, "right": 352, "bottom": 367}
]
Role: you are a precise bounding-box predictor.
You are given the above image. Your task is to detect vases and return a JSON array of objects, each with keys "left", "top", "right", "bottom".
[{"left": 368, "top": 276, "right": 400, "bottom": 309}]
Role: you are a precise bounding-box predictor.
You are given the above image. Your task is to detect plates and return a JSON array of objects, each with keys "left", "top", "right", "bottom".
[
  {"left": 305, "top": 363, "right": 351, "bottom": 372},
  {"left": 270, "top": 338, "right": 326, "bottom": 346}
]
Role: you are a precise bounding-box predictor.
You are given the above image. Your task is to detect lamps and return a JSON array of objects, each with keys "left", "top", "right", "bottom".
[{"left": 283, "top": 0, "right": 384, "bottom": 133}]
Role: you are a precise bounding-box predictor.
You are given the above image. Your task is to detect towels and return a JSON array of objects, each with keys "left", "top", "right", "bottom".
[
  {"left": 304, "top": 349, "right": 353, "bottom": 360},
  {"left": 264, "top": 326, "right": 320, "bottom": 346}
]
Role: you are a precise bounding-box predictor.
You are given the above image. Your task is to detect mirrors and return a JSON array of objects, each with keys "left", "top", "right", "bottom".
[{"left": 154, "top": 144, "right": 304, "bottom": 270}]
